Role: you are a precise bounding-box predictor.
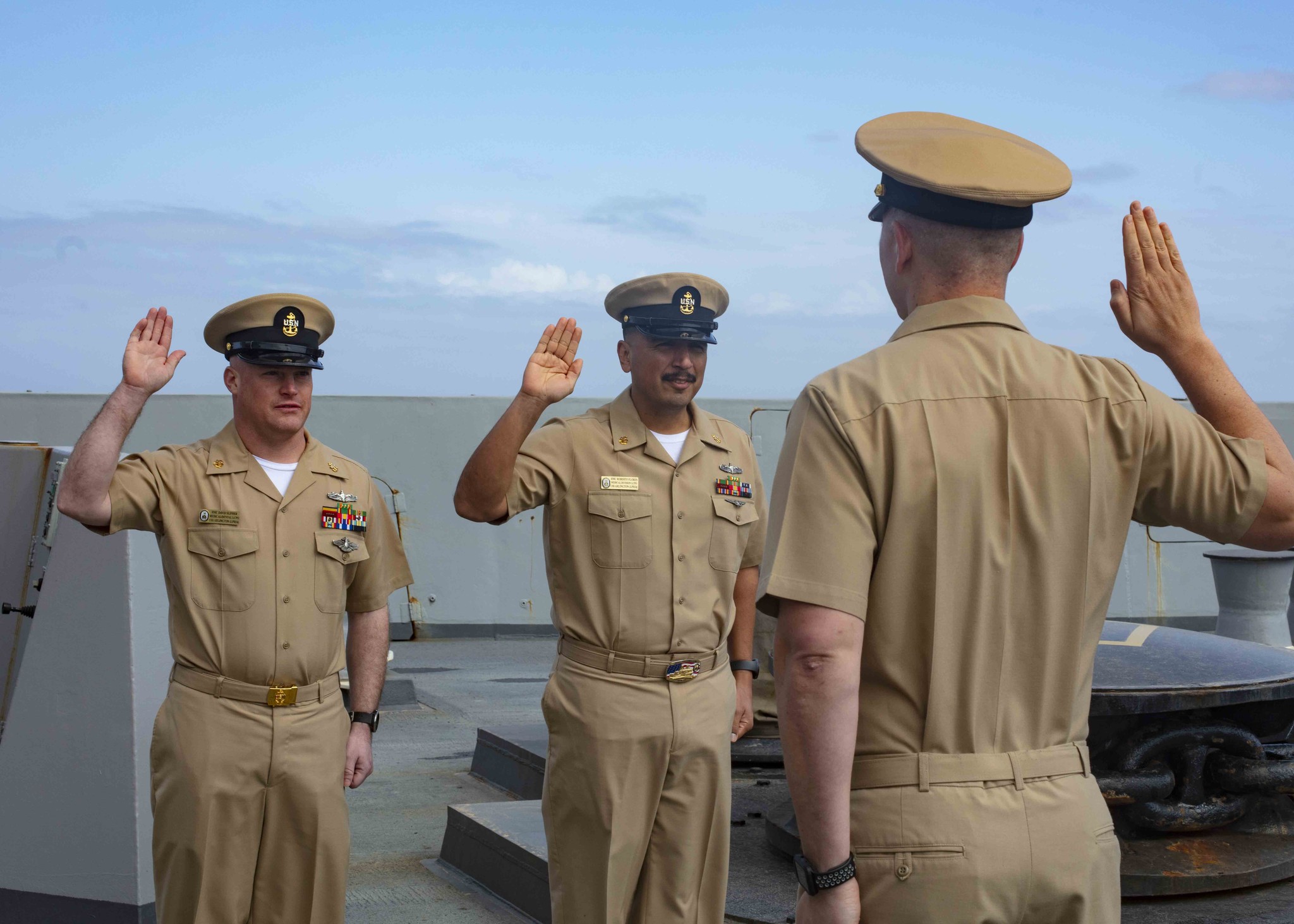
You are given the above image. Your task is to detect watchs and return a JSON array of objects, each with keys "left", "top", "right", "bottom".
[
  {"left": 730, "top": 658, "right": 760, "bottom": 679},
  {"left": 348, "top": 710, "right": 380, "bottom": 733},
  {"left": 793, "top": 850, "right": 857, "bottom": 897}
]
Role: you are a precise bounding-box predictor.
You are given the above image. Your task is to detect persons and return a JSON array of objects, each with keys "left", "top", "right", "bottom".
[
  {"left": 455, "top": 273, "right": 767, "bottom": 924},
  {"left": 755, "top": 113, "right": 1294, "bottom": 924},
  {"left": 55, "top": 292, "right": 415, "bottom": 924}
]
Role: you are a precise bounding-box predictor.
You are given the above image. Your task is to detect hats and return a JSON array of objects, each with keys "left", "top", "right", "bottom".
[
  {"left": 854, "top": 113, "right": 1072, "bottom": 231},
  {"left": 603, "top": 272, "right": 732, "bottom": 344},
  {"left": 202, "top": 292, "right": 336, "bottom": 369}
]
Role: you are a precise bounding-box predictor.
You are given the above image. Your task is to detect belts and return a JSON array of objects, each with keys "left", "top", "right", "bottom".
[
  {"left": 560, "top": 639, "right": 726, "bottom": 681},
  {"left": 172, "top": 662, "right": 340, "bottom": 707},
  {"left": 848, "top": 746, "right": 1089, "bottom": 790}
]
